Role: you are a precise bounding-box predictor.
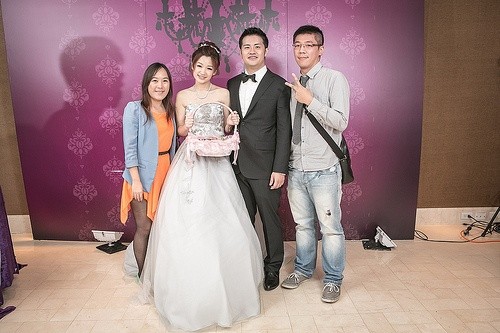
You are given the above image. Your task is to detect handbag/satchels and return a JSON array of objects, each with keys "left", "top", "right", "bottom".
[{"left": 339, "top": 134, "right": 354, "bottom": 185}]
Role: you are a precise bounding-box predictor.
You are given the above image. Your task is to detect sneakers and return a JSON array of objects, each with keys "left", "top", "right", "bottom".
[
  {"left": 282, "top": 272, "right": 309, "bottom": 288},
  {"left": 321, "top": 283, "right": 341, "bottom": 303}
]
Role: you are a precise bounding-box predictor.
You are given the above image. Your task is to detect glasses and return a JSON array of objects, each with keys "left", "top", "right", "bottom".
[{"left": 292, "top": 41, "right": 321, "bottom": 49}]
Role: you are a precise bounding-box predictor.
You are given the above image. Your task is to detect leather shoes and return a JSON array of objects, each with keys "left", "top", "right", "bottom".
[{"left": 263, "top": 270, "right": 279, "bottom": 291}]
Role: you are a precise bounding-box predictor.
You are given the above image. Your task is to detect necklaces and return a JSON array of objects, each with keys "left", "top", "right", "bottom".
[{"left": 193, "top": 82, "right": 212, "bottom": 100}]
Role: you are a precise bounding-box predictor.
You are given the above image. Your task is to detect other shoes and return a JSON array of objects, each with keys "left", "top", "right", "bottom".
[{"left": 136, "top": 272, "right": 155, "bottom": 302}]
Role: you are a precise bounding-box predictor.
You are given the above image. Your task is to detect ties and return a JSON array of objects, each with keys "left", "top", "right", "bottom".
[{"left": 292, "top": 76, "right": 310, "bottom": 145}]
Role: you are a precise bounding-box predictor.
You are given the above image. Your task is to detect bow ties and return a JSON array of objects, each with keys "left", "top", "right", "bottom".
[{"left": 241, "top": 72, "right": 257, "bottom": 83}]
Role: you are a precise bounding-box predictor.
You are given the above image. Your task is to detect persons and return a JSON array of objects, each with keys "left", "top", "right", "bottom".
[
  {"left": 133, "top": 41, "right": 265, "bottom": 332},
  {"left": 280, "top": 25, "right": 350, "bottom": 304},
  {"left": 226, "top": 27, "right": 292, "bottom": 291},
  {"left": 122, "top": 62, "right": 177, "bottom": 294}
]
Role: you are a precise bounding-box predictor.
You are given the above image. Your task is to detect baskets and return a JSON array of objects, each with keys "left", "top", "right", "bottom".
[{"left": 186, "top": 101, "right": 241, "bottom": 165}]
